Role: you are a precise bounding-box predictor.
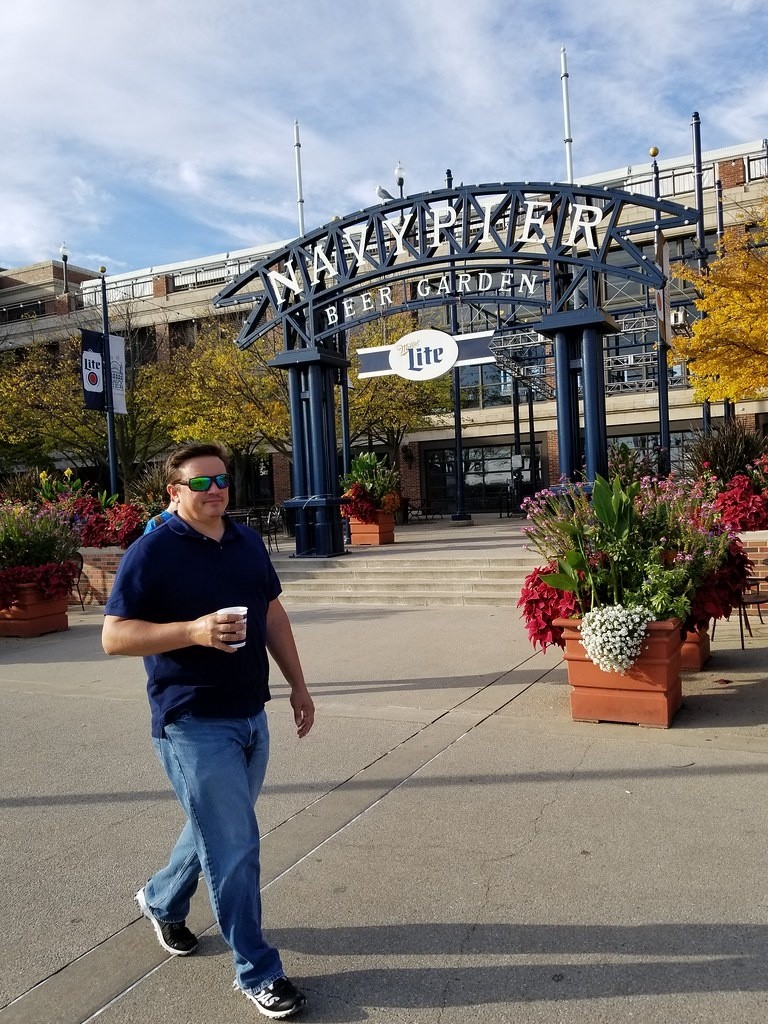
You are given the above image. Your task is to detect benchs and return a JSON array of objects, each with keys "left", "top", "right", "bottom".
[{"left": 408, "top": 508, "right": 444, "bottom": 522}]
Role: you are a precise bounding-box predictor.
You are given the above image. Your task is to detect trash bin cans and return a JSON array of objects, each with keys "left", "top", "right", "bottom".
[{"left": 396, "top": 498, "right": 410, "bottom": 525}]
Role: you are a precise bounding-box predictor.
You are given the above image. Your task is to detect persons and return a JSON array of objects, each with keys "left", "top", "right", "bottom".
[{"left": 100, "top": 445, "right": 317, "bottom": 1019}]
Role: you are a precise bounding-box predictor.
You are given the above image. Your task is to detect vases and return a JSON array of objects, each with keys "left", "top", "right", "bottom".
[
  {"left": 346, "top": 509, "right": 395, "bottom": 545},
  {"left": 681, "top": 618, "right": 711, "bottom": 672},
  {"left": 550, "top": 614, "right": 685, "bottom": 728},
  {"left": 0, "top": 583, "right": 68, "bottom": 638}
]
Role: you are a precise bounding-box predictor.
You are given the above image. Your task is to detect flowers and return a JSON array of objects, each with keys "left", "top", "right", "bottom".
[
  {"left": 329, "top": 448, "right": 402, "bottom": 525},
  {"left": 0, "top": 496, "right": 86, "bottom": 607},
  {"left": 516, "top": 418, "right": 767, "bottom": 655}
]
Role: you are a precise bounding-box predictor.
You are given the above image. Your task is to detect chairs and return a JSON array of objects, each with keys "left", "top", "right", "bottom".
[
  {"left": 243, "top": 505, "right": 281, "bottom": 556},
  {"left": 735, "top": 579, "right": 768, "bottom": 629}
]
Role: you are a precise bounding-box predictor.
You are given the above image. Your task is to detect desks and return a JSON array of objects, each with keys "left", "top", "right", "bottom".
[
  {"left": 226, "top": 510, "right": 272, "bottom": 533},
  {"left": 406, "top": 497, "right": 441, "bottom": 519}
]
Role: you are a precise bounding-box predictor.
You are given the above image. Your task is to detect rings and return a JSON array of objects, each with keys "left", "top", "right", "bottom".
[{"left": 220, "top": 634, "right": 223, "bottom": 641}]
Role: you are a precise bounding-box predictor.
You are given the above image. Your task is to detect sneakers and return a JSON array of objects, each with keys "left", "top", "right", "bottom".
[
  {"left": 134, "top": 887, "right": 198, "bottom": 956},
  {"left": 232, "top": 971, "right": 306, "bottom": 1020}
]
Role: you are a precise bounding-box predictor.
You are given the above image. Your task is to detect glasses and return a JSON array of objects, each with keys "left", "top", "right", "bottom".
[{"left": 172, "top": 474, "right": 229, "bottom": 491}]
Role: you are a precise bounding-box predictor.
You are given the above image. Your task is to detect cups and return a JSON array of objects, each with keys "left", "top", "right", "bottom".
[{"left": 216, "top": 606, "right": 248, "bottom": 648}]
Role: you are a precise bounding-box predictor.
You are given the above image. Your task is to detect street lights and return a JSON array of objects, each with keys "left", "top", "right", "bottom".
[
  {"left": 59, "top": 241, "right": 70, "bottom": 294},
  {"left": 395, "top": 160, "right": 409, "bottom": 228}
]
